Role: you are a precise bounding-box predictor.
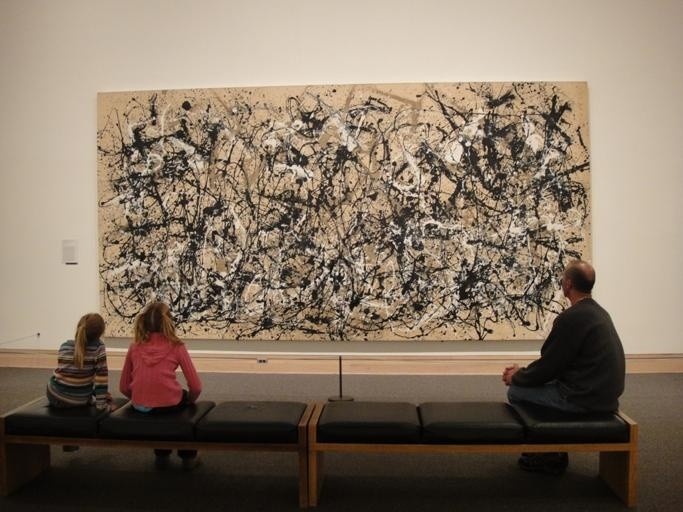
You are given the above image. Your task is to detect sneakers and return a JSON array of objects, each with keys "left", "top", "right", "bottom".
[
  {"left": 154, "top": 454, "right": 175, "bottom": 469},
  {"left": 63, "top": 450, "right": 82, "bottom": 462},
  {"left": 517, "top": 452, "right": 570, "bottom": 474},
  {"left": 183, "top": 448, "right": 205, "bottom": 471}
]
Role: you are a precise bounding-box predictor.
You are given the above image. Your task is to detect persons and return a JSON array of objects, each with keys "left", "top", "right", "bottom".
[
  {"left": 499, "top": 257, "right": 625, "bottom": 475},
  {"left": 116, "top": 300, "right": 202, "bottom": 469},
  {"left": 44, "top": 311, "right": 118, "bottom": 460}
]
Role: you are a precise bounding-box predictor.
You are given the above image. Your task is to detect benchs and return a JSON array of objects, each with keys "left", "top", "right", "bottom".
[
  {"left": 0, "top": 397, "right": 314, "bottom": 509},
  {"left": 308, "top": 402, "right": 639, "bottom": 508}
]
